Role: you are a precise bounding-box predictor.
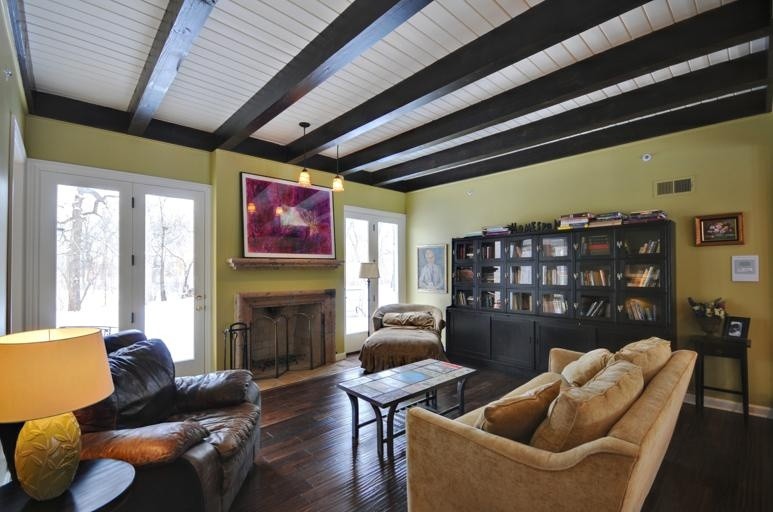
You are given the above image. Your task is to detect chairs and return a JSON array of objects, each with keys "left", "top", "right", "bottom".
[{"left": 73, "top": 330, "right": 262, "bottom": 510}]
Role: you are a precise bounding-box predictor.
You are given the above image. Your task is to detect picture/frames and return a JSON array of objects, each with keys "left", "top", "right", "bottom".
[
  {"left": 722, "top": 316, "right": 750, "bottom": 342},
  {"left": 416, "top": 243, "right": 449, "bottom": 294},
  {"left": 694, "top": 211, "right": 745, "bottom": 247},
  {"left": 239, "top": 170, "right": 336, "bottom": 260}
]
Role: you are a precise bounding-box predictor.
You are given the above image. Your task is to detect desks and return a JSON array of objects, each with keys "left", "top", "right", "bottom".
[
  {"left": 686, "top": 335, "right": 751, "bottom": 428},
  {"left": 0, "top": 458, "right": 135, "bottom": 511}
]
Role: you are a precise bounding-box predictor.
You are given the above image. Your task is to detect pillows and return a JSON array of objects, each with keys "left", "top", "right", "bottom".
[
  {"left": 381, "top": 311, "right": 434, "bottom": 329},
  {"left": 529, "top": 358, "right": 644, "bottom": 453},
  {"left": 475, "top": 379, "right": 562, "bottom": 445},
  {"left": 561, "top": 348, "right": 614, "bottom": 387},
  {"left": 613, "top": 337, "right": 671, "bottom": 386},
  {"left": 96, "top": 340, "right": 175, "bottom": 422}
]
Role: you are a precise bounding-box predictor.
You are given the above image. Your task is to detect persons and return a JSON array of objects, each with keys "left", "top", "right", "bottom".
[
  {"left": 729, "top": 324, "right": 741, "bottom": 337},
  {"left": 419, "top": 248, "right": 444, "bottom": 289}
]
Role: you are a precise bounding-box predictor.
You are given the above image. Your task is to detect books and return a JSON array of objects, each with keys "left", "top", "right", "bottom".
[{"left": 456, "top": 209, "right": 667, "bottom": 321}]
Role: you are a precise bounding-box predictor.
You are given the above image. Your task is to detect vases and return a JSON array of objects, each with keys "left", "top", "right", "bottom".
[{"left": 699, "top": 318, "right": 721, "bottom": 340}]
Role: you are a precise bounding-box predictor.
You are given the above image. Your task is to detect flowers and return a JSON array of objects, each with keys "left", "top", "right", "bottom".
[{"left": 688, "top": 297, "right": 727, "bottom": 320}]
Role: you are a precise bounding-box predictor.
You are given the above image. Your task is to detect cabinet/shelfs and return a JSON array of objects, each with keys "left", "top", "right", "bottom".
[
  {"left": 446, "top": 306, "right": 491, "bottom": 371},
  {"left": 598, "top": 322, "right": 676, "bottom": 351},
  {"left": 451, "top": 219, "right": 676, "bottom": 325},
  {"left": 535, "top": 315, "right": 597, "bottom": 376},
  {"left": 490, "top": 310, "right": 535, "bottom": 377}
]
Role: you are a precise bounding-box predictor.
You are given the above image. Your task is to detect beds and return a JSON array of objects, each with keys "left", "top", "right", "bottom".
[{"left": 359, "top": 303, "right": 447, "bottom": 373}]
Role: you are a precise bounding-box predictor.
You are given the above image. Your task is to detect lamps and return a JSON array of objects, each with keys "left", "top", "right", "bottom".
[
  {"left": 330, "top": 144, "right": 344, "bottom": 191},
  {"left": 0, "top": 327, "right": 115, "bottom": 501},
  {"left": 298, "top": 121, "right": 312, "bottom": 187},
  {"left": 359, "top": 262, "right": 381, "bottom": 337}
]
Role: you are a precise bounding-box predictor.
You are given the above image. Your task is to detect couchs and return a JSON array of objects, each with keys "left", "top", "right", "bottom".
[{"left": 406, "top": 336, "right": 698, "bottom": 512}]
